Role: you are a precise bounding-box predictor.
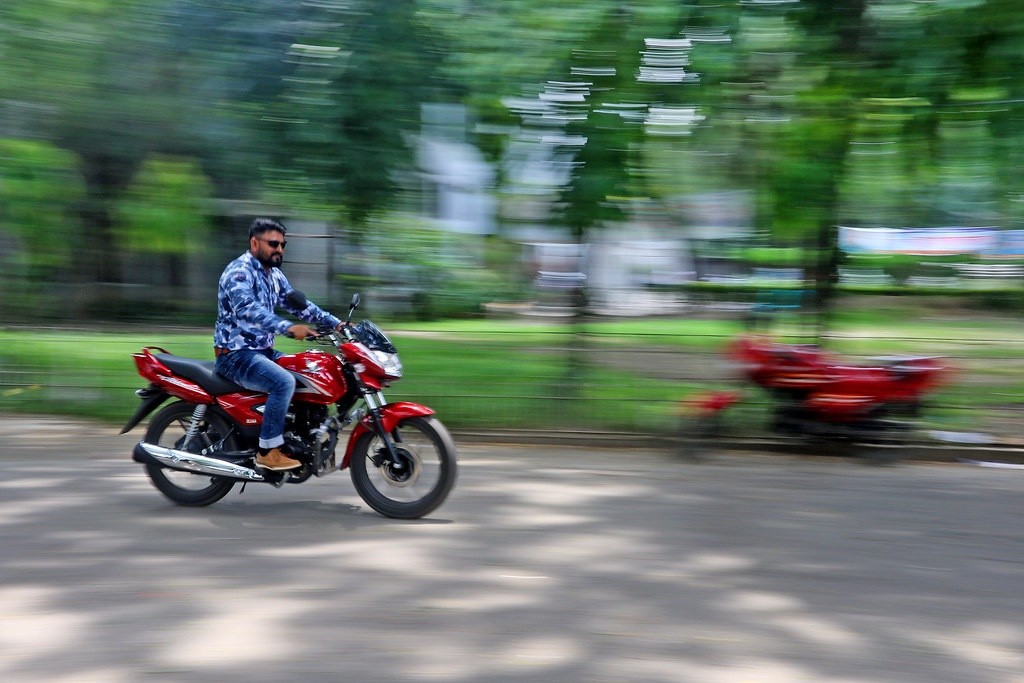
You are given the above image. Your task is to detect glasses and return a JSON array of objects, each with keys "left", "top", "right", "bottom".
[{"left": 255, "top": 236, "right": 287, "bottom": 249}]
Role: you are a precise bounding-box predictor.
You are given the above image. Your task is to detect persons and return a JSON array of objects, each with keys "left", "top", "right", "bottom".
[{"left": 213, "top": 218, "right": 355, "bottom": 471}]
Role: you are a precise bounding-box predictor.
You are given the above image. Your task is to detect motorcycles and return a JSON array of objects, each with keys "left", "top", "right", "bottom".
[
  {"left": 727, "top": 339, "right": 953, "bottom": 455},
  {"left": 118, "top": 291, "right": 457, "bottom": 520}
]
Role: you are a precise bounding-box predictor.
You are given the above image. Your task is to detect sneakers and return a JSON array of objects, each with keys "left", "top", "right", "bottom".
[{"left": 255, "top": 448, "right": 302, "bottom": 472}]
